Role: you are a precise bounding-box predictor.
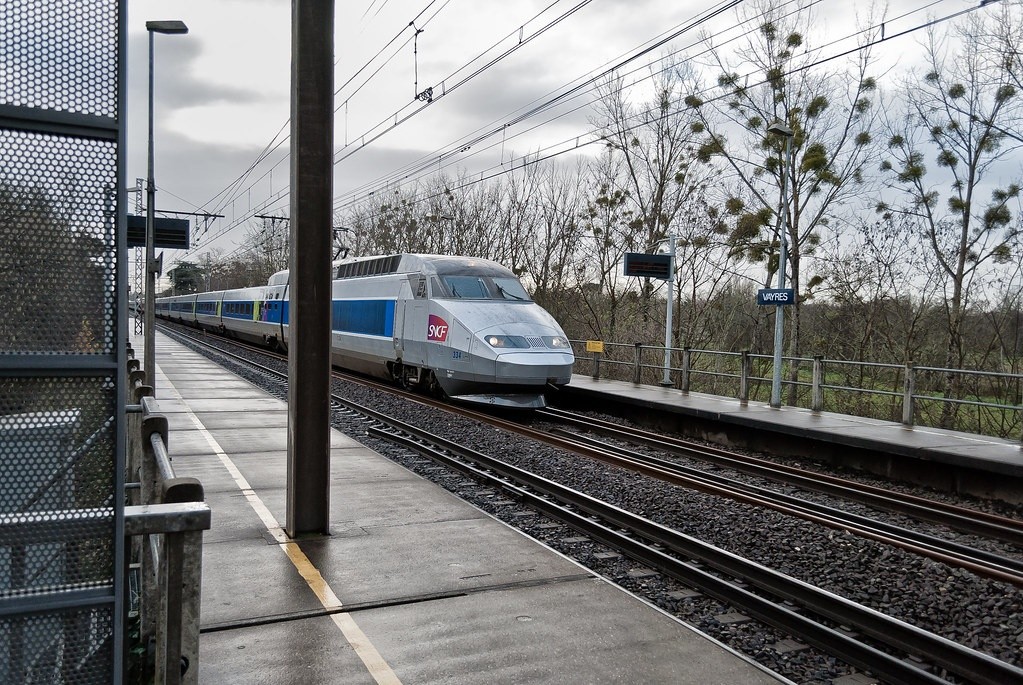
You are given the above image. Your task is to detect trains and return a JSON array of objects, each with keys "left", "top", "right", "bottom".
[{"left": 129, "top": 252, "right": 575, "bottom": 412}]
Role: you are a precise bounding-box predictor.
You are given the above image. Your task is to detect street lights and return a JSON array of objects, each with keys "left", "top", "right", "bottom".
[{"left": 145, "top": 19, "right": 189, "bottom": 396}]
